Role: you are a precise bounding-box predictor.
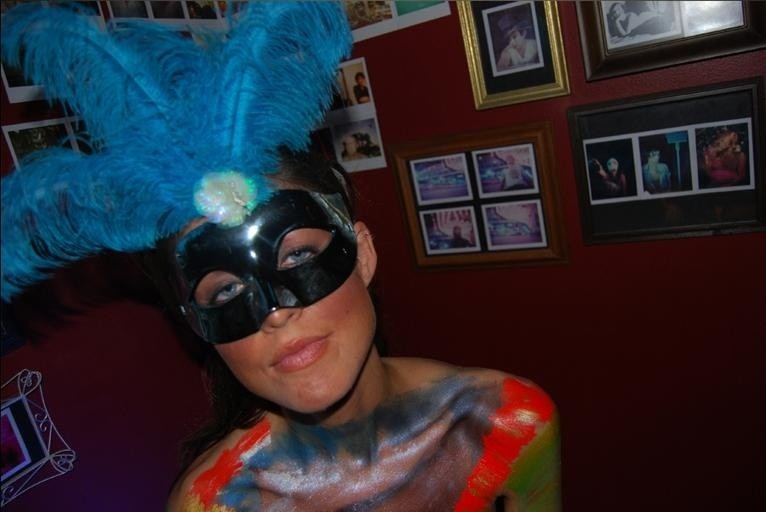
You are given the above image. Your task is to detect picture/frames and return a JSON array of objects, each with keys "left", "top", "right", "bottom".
[
  {"left": 566, "top": 78, "right": 765, "bottom": 243},
  {"left": 572, "top": 2, "right": 762, "bottom": 84},
  {"left": 398, "top": 119, "right": 572, "bottom": 267},
  {"left": 0, "top": 369, "right": 75, "bottom": 512},
  {"left": 454, "top": 0, "right": 575, "bottom": 111}
]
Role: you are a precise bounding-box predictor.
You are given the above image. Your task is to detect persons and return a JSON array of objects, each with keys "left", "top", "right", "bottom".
[
  {"left": 352, "top": 70, "right": 370, "bottom": 105},
  {"left": 344, "top": 134, "right": 371, "bottom": 163},
  {"left": 497, "top": 154, "right": 533, "bottom": 192},
  {"left": 590, "top": 154, "right": 627, "bottom": 198},
  {"left": 496, "top": 15, "right": 539, "bottom": 71},
  {"left": 609, "top": 2, "right": 669, "bottom": 41},
  {"left": 157, "top": 145, "right": 566, "bottom": 511},
  {"left": 642, "top": 146, "right": 673, "bottom": 195}
]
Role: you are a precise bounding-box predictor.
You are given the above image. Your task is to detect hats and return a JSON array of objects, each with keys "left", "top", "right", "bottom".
[{"left": 492, "top": 14, "right": 533, "bottom": 42}]
